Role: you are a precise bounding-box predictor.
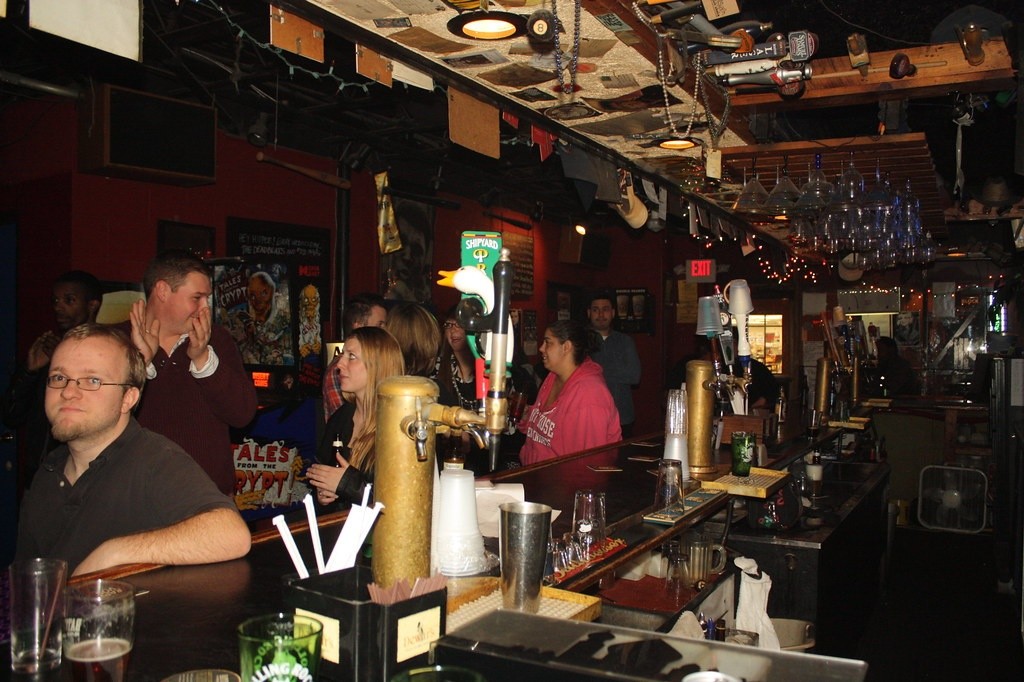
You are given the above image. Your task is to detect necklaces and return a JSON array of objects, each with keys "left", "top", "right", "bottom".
[{"left": 450, "top": 355, "right": 478, "bottom": 404}]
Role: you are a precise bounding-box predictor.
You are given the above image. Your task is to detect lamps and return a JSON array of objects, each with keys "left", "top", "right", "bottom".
[
  {"left": 607, "top": 168, "right": 648, "bottom": 230},
  {"left": 345, "top": 143, "right": 371, "bottom": 174},
  {"left": 530, "top": 201, "right": 546, "bottom": 224},
  {"left": 247, "top": 110, "right": 270, "bottom": 148},
  {"left": 426, "top": 159, "right": 449, "bottom": 191},
  {"left": 574, "top": 217, "right": 598, "bottom": 236},
  {"left": 476, "top": 186, "right": 503, "bottom": 208}
]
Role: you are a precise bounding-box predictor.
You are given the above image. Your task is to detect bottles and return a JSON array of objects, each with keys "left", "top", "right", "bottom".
[
  {"left": 328, "top": 432, "right": 344, "bottom": 468},
  {"left": 697, "top": 612, "right": 726, "bottom": 641},
  {"left": 813, "top": 449, "right": 821, "bottom": 465},
  {"left": 774, "top": 383, "right": 787, "bottom": 423},
  {"left": 442, "top": 426, "right": 467, "bottom": 470}
]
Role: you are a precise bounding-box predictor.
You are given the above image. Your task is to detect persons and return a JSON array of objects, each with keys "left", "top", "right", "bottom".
[
  {"left": 722, "top": 357, "right": 780, "bottom": 413},
  {"left": 0, "top": 249, "right": 253, "bottom": 591},
  {"left": 306, "top": 289, "right": 641, "bottom": 515},
  {"left": 872, "top": 336, "right": 915, "bottom": 394}
]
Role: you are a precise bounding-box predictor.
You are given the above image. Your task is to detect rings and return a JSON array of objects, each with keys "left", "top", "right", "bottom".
[
  {"left": 145, "top": 330, "right": 151, "bottom": 334},
  {"left": 317, "top": 491, "right": 324, "bottom": 496}
]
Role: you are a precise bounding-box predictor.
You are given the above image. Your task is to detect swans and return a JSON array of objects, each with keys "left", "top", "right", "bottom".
[{"left": 438, "top": 265, "right": 515, "bottom": 365}]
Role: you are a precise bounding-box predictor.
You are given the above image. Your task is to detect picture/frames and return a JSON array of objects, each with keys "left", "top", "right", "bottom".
[
  {"left": 155, "top": 218, "right": 216, "bottom": 266},
  {"left": 377, "top": 196, "right": 437, "bottom": 306}
]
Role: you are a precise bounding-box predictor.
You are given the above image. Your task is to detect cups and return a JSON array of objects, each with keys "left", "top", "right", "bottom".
[
  {"left": 652, "top": 389, "right": 689, "bottom": 515},
  {"left": 806, "top": 409, "right": 823, "bottom": 440},
  {"left": 727, "top": 279, "right": 754, "bottom": 315},
  {"left": 160, "top": 612, "right": 323, "bottom": 682},
  {"left": 733, "top": 432, "right": 756, "bottom": 476},
  {"left": 428, "top": 469, "right": 487, "bottom": 576},
  {"left": 660, "top": 529, "right": 728, "bottom": 596},
  {"left": 543, "top": 488, "right": 606, "bottom": 587},
  {"left": 8, "top": 557, "right": 68, "bottom": 673},
  {"left": 509, "top": 391, "right": 528, "bottom": 423},
  {"left": 681, "top": 670, "right": 739, "bottom": 682},
  {"left": 390, "top": 665, "right": 486, "bottom": 682},
  {"left": 806, "top": 464, "right": 823, "bottom": 497},
  {"left": 696, "top": 296, "right": 724, "bottom": 336},
  {"left": 61, "top": 579, "right": 135, "bottom": 663},
  {"left": 497, "top": 502, "right": 552, "bottom": 613}
]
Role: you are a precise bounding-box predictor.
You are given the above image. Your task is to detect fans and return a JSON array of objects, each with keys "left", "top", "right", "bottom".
[{"left": 917, "top": 463, "right": 989, "bottom": 534}]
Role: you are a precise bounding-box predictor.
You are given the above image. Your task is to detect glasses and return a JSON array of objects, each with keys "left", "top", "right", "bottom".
[
  {"left": 48, "top": 373, "right": 134, "bottom": 391},
  {"left": 443, "top": 322, "right": 461, "bottom": 328}
]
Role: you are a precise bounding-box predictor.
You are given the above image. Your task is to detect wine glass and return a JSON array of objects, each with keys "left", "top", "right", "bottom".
[{"left": 729, "top": 147, "right": 937, "bottom": 271}]
